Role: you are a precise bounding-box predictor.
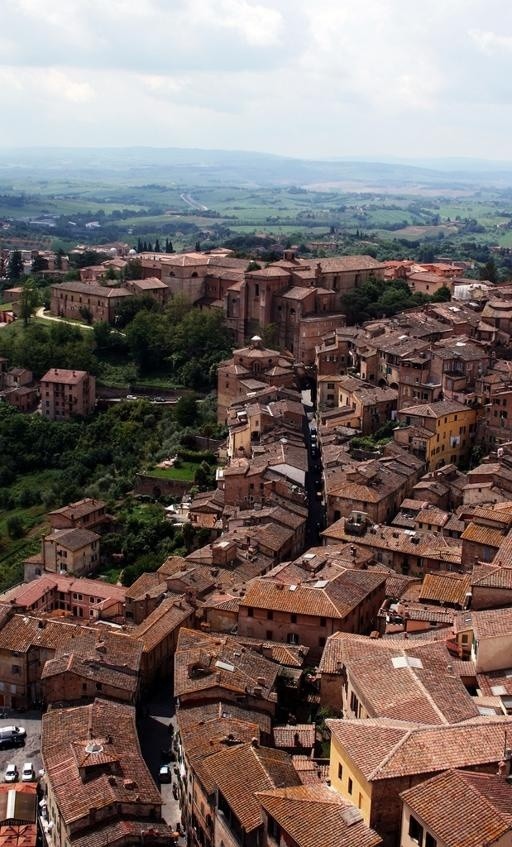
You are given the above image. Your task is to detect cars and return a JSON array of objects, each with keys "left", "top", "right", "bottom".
[
  {"left": 158, "top": 764, "right": 171, "bottom": 780},
  {"left": 0, "top": 726, "right": 26, "bottom": 751},
  {"left": 4, "top": 763, "right": 34, "bottom": 783}
]
